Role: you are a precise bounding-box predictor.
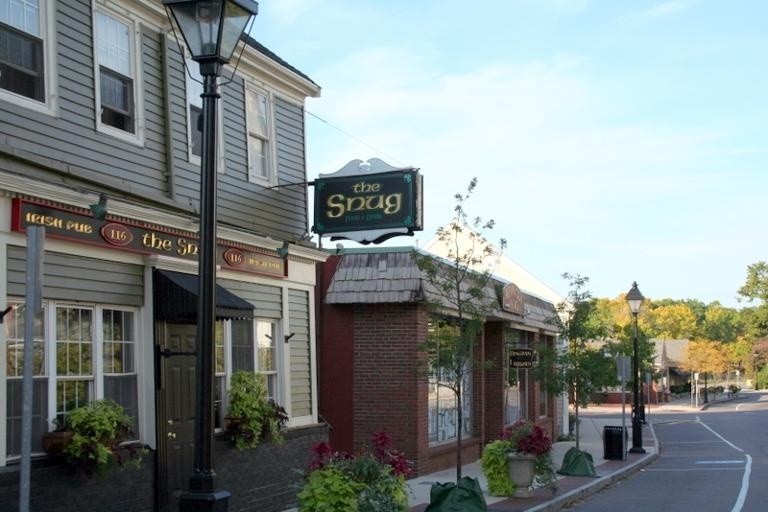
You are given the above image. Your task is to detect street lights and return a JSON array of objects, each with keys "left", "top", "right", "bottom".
[
  {"left": 625, "top": 281, "right": 647, "bottom": 454},
  {"left": 162, "top": 0, "right": 259, "bottom": 512}
]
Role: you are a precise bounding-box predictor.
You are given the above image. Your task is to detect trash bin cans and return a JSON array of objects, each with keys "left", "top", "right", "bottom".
[{"left": 603, "top": 426, "right": 628, "bottom": 461}]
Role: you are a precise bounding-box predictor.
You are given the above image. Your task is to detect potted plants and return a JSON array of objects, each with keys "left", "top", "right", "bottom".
[
  {"left": 222, "top": 369, "right": 287, "bottom": 452},
  {"left": 482, "top": 420, "right": 568, "bottom": 499},
  {"left": 41, "top": 399, "right": 148, "bottom": 481}
]
